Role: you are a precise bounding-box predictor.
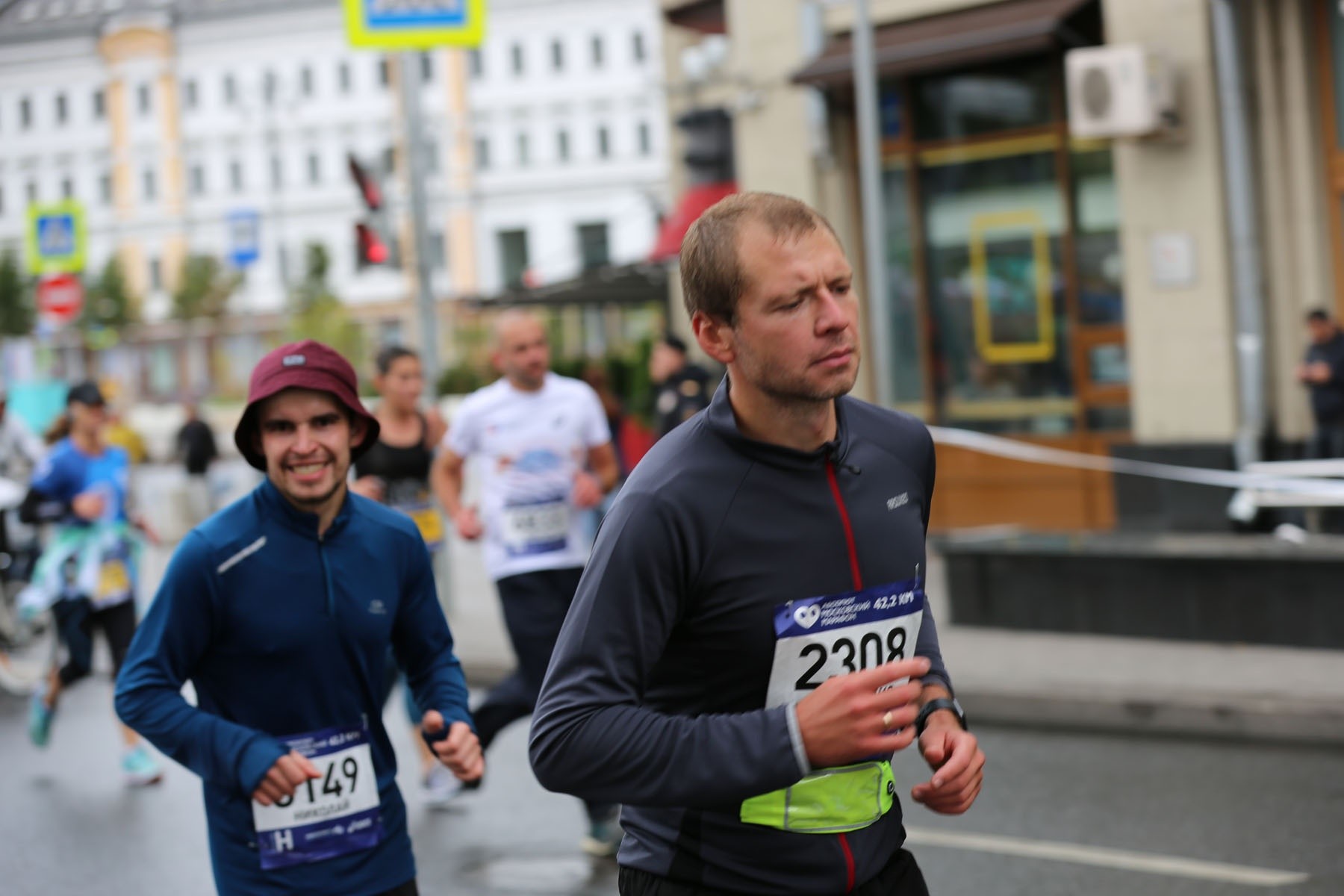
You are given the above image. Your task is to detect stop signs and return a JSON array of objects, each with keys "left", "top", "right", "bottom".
[{"left": 34, "top": 271, "right": 82, "bottom": 323}]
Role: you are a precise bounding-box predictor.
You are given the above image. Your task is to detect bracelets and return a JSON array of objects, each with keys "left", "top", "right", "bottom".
[{"left": 911, "top": 696, "right": 970, "bottom": 736}]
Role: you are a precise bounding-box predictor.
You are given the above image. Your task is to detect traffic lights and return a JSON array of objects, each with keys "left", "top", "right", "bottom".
[
  {"left": 342, "top": 147, "right": 407, "bottom": 269},
  {"left": 676, "top": 107, "right": 735, "bottom": 187}
]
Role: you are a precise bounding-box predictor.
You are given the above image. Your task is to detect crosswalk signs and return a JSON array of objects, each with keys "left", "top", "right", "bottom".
[{"left": 34, "top": 215, "right": 75, "bottom": 258}]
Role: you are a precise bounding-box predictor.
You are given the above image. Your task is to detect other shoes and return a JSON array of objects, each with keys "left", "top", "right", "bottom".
[
  {"left": 123, "top": 750, "right": 164, "bottom": 786},
  {"left": 26, "top": 688, "right": 55, "bottom": 744}
]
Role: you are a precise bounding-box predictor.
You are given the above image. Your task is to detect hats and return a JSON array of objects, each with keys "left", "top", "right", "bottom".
[
  {"left": 234, "top": 340, "right": 379, "bottom": 471},
  {"left": 68, "top": 384, "right": 104, "bottom": 406}
]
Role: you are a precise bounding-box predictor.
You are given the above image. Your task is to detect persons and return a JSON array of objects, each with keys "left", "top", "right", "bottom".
[
  {"left": 0, "top": 192, "right": 985, "bottom": 896},
  {"left": 110, "top": 339, "right": 485, "bottom": 896},
  {"left": 529, "top": 190, "right": 984, "bottom": 896},
  {"left": 1269, "top": 310, "right": 1344, "bottom": 545}
]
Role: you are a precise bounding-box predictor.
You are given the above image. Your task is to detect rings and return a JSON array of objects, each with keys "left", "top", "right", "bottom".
[{"left": 882, "top": 710, "right": 892, "bottom": 736}]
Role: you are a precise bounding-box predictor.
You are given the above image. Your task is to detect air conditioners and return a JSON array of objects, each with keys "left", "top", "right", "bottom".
[{"left": 1065, "top": 46, "right": 1184, "bottom": 139}]
